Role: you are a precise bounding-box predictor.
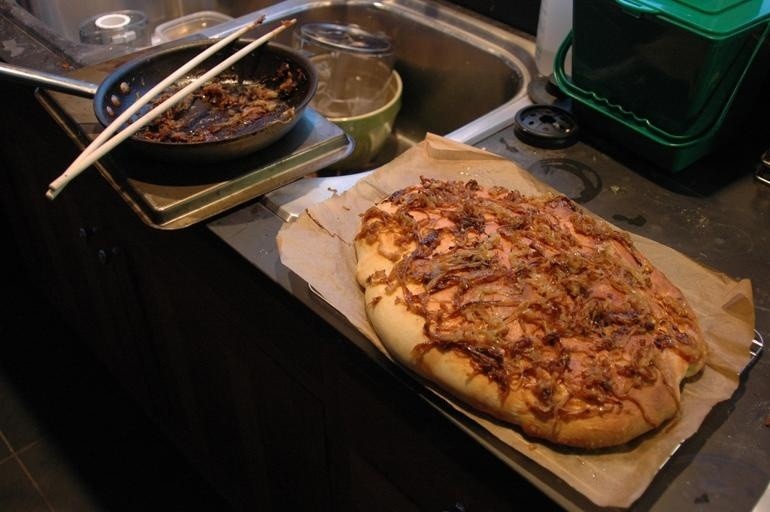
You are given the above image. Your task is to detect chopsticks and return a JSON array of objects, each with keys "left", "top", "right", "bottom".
[{"left": 45, "top": 17, "right": 299, "bottom": 201}]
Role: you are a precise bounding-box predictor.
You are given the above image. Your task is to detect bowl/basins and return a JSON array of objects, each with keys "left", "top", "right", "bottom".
[{"left": 93, "top": 38, "right": 319, "bottom": 165}]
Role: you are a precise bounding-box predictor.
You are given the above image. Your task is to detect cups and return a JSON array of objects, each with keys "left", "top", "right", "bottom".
[
  {"left": 291, "top": 21, "right": 396, "bottom": 117},
  {"left": 306, "top": 54, "right": 403, "bottom": 173}
]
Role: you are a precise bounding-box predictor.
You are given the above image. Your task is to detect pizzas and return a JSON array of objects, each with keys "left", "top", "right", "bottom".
[{"left": 354, "top": 175, "right": 708, "bottom": 449}]
